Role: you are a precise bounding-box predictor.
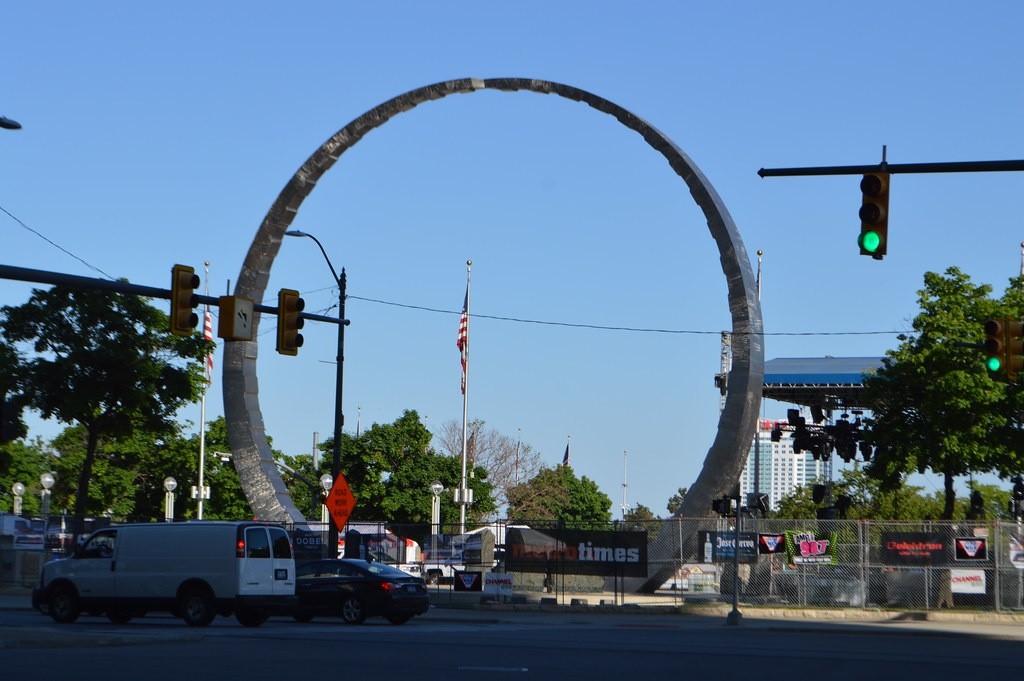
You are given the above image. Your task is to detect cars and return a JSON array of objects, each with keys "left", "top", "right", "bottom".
[{"left": 294, "top": 559, "right": 432, "bottom": 625}]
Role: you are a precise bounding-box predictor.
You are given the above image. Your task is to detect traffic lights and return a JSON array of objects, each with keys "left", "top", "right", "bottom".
[
  {"left": 277, "top": 288, "right": 305, "bottom": 356},
  {"left": 983, "top": 318, "right": 1005, "bottom": 376},
  {"left": 172, "top": 265, "right": 200, "bottom": 338},
  {"left": 1006, "top": 317, "right": 1024, "bottom": 376},
  {"left": 857, "top": 172, "right": 890, "bottom": 256}
]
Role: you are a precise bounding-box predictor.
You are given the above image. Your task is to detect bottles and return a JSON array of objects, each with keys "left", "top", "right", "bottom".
[{"left": 704, "top": 533, "right": 713, "bottom": 563}]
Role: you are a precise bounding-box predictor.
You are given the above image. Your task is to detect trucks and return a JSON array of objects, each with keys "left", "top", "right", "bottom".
[{"left": 38, "top": 523, "right": 297, "bottom": 627}]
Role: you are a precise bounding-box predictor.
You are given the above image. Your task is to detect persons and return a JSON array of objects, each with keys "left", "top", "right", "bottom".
[{"left": 100, "top": 551, "right": 113, "bottom": 557}]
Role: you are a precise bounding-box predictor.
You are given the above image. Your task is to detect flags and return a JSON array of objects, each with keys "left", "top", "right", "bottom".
[
  {"left": 202, "top": 291, "right": 215, "bottom": 387},
  {"left": 456, "top": 279, "right": 469, "bottom": 396}
]
